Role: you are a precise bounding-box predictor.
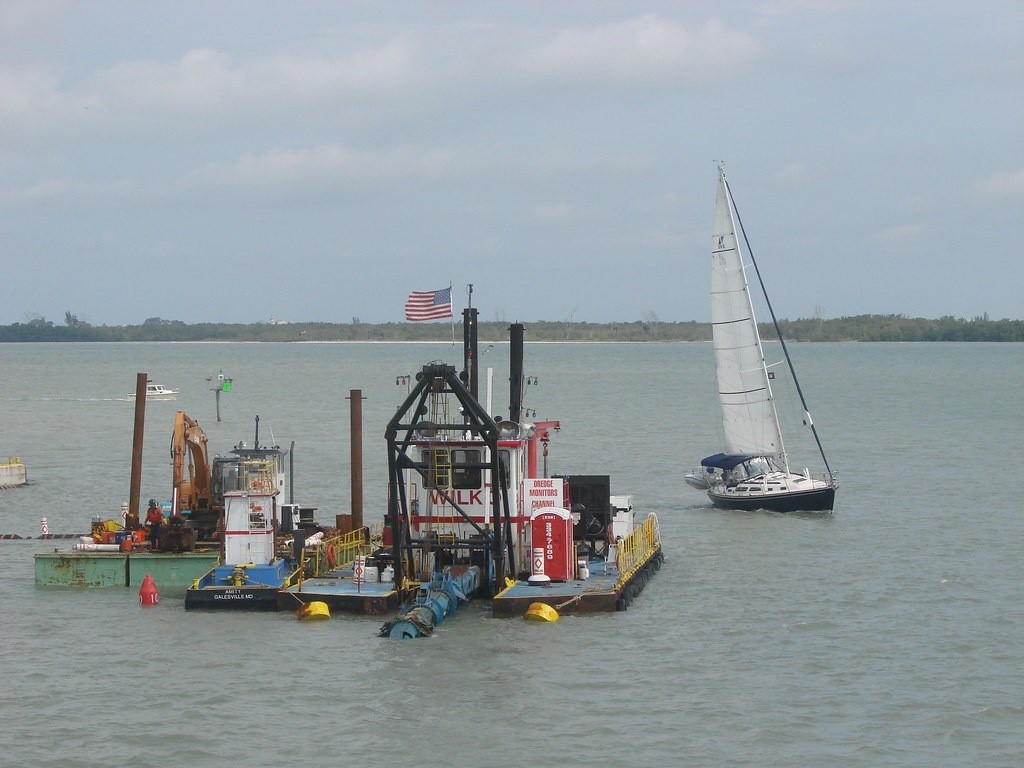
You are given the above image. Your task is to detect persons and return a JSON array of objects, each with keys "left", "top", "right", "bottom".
[{"left": 145, "top": 499, "right": 163, "bottom": 552}]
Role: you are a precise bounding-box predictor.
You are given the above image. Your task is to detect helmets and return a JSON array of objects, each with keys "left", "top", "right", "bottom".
[{"left": 148, "top": 499, "right": 158, "bottom": 506}]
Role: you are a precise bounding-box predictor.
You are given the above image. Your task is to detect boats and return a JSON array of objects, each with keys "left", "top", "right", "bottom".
[{"left": 126, "top": 380, "right": 179, "bottom": 400}]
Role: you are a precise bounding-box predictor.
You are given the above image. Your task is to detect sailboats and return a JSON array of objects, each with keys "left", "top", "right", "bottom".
[{"left": 685, "top": 166, "right": 839, "bottom": 514}]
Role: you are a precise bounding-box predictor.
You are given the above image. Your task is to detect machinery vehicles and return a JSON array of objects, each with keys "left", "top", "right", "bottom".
[{"left": 158, "top": 410, "right": 242, "bottom": 553}]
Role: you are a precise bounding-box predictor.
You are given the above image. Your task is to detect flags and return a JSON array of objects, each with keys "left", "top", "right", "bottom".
[{"left": 405, "top": 287, "right": 453, "bottom": 321}]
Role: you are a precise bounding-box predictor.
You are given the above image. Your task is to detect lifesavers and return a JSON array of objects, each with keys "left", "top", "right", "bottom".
[
  {"left": 616, "top": 552, "right": 664, "bottom": 611},
  {"left": 328, "top": 544, "right": 335, "bottom": 558}
]
